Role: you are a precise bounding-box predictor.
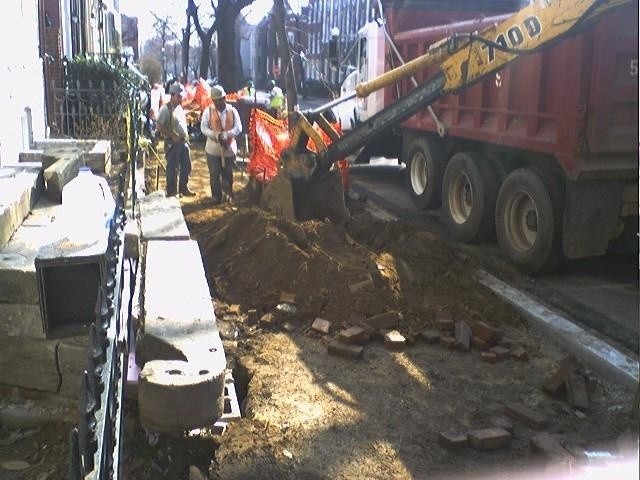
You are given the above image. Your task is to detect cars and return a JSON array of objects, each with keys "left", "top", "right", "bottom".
[{"left": 166, "top": 72, "right": 219, "bottom": 88}]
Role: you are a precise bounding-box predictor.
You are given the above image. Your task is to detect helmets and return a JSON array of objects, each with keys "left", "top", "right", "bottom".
[
  {"left": 268, "top": 79, "right": 276, "bottom": 86},
  {"left": 209, "top": 85, "right": 226, "bottom": 100},
  {"left": 247, "top": 80, "right": 255, "bottom": 88},
  {"left": 168, "top": 81, "right": 185, "bottom": 94}
]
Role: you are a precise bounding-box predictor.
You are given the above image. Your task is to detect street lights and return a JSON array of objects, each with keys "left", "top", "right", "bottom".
[
  {"left": 161, "top": 47, "right": 166, "bottom": 88},
  {"left": 332, "top": 26, "right": 341, "bottom": 59}
]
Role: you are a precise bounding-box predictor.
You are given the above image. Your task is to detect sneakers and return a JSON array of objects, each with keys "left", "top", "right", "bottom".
[
  {"left": 166, "top": 190, "right": 183, "bottom": 199},
  {"left": 178, "top": 188, "right": 197, "bottom": 197}
]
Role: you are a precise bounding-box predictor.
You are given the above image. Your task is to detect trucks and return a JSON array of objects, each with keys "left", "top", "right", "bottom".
[{"left": 328, "top": 0, "right": 639, "bottom": 277}]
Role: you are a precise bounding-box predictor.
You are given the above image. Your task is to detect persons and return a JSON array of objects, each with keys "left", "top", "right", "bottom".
[
  {"left": 156, "top": 85, "right": 196, "bottom": 198},
  {"left": 136, "top": 72, "right": 285, "bottom": 149},
  {"left": 200, "top": 84, "right": 243, "bottom": 206}
]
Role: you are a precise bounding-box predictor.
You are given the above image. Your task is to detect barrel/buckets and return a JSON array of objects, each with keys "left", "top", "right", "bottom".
[{"left": 60, "top": 166, "right": 116, "bottom": 249}]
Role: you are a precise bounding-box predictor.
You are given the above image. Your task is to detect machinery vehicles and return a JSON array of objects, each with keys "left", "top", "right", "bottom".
[{"left": 258, "top": 0, "right": 638, "bottom": 226}]
